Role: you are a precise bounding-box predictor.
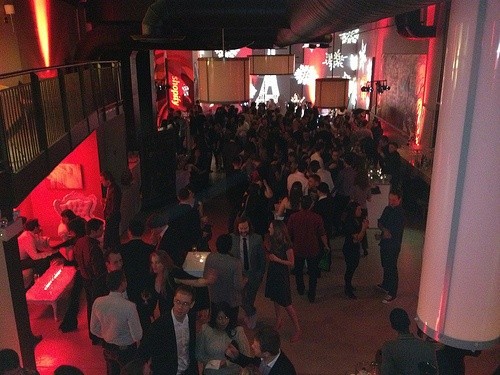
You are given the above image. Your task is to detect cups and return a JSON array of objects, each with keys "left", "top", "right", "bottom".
[{"left": 192, "top": 247, "right": 197, "bottom": 256}]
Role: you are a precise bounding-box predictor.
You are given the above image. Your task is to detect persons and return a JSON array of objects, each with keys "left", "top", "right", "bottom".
[
  {"left": 185, "top": 186, "right": 208, "bottom": 231},
  {"left": 100, "top": 172, "right": 122, "bottom": 253},
  {"left": 51, "top": 208, "right": 109, "bottom": 345},
  {"left": 17, "top": 217, "right": 52, "bottom": 276},
  {"left": 341, "top": 202, "right": 369, "bottom": 298},
  {"left": 90, "top": 218, "right": 250, "bottom": 375},
  {"left": 378, "top": 307, "right": 465, "bottom": 375},
  {"left": 0, "top": 349, "right": 84, "bottom": 375},
  {"left": 376, "top": 190, "right": 406, "bottom": 304},
  {"left": 224, "top": 326, "right": 296, "bottom": 375},
  {"left": 228, "top": 217, "right": 301, "bottom": 341},
  {"left": 168, "top": 99, "right": 401, "bottom": 241},
  {"left": 286, "top": 196, "right": 330, "bottom": 304},
  {"left": 312, "top": 182, "right": 333, "bottom": 273},
  {"left": 167, "top": 189, "right": 209, "bottom": 265},
  {"left": 121, "top": 152, "right": 159, "bottom": 221}
]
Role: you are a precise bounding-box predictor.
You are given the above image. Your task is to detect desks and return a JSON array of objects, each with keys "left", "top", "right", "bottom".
[
  {"left": 181, "top": 252, "right": 212, "bottom": 278},
  {"left": 26, "top": 265, "right": 78, "bottom": 323}
]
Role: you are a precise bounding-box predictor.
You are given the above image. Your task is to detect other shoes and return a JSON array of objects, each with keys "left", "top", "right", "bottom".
[
  {"left": 275, "top": 319, "right": 284, "bottom": 331},
  {"left": 290, "top": 329, "right": 304, "bottom": 343},
  {"left": 343, "top": 291, "right": 358, "bottom": 300},
  {"left": 383, "top": 294, "right": 396, "bottom": 304},
  {"left": 307, "top": 292, "right": 316, "bottom": 303},
  {"left": 87, "top": 334, "right": 105, "bottom": 345},
  {"left": 298, "top": 289, "right": 304, "bottom": 295},
  {"left": 376, "top": 282, "right": 389, "bottom": 293}
]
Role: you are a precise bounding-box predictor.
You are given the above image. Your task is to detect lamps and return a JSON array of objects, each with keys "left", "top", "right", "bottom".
[
  {"left": 247, "top": 43, "right": 296, "bottom": 76},
  {"left": 196, "top": 27, "right": 250, "bottom": 104},
  {"left": 361, "top": 79, "right": 391, "bottom": 94},
  {"left": 314, "top": 32, "right": 349, "bottom": 109}
]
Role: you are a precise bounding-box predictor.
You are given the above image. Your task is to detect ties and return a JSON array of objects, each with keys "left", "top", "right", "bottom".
[{"left": 241, "top": 238, "right": 250, "bottom": 273}]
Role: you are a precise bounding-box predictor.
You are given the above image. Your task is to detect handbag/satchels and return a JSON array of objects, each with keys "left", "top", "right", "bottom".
[{"left": 314, "top": 250, "right": 332, "bottom": 273}]
